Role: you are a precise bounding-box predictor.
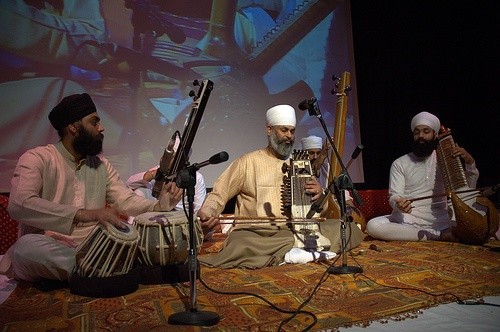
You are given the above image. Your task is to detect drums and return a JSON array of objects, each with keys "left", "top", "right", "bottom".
[
  {"left": 74, "top": 218, "right": 142, "bottom": 280},
  {"left": 132, "top": 211, "right": 204, "bottom": 270}
]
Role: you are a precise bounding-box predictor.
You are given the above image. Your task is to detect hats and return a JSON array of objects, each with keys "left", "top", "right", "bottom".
[
  {"left": 48, "top": 93, "right": 97, "bottom": 131},
  {"left": 302, "top": 135, "right": 322, "bottom": 149},
  {"left": 266, "top": 105, "right": 297, "bottom": 127},
  {"left": 411, "top": 112, "right": 440, "bottom": 140},
  {"left": 167, "top": 136, "right": 193, "bottom": 158}
]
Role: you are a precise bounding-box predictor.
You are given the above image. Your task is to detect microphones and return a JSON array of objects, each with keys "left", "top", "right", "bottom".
[
  {"left": 94, "top": 41, "right": 188, "bottom": 79},
  {"left": 306, "top": 188, "right": 329, "bottom": 219},
  {"left": 299, "top": 97, "right": 317, "bottom": 110},
  {"left": 152, "top": 134, "right": 177, "bottom": 198}
]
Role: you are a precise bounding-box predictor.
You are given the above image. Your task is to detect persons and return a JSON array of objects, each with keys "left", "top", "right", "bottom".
[
  {"left": 301, "top": 136, "right": 355, "bottom": 209},
  {"left": 366, "top": 112, "right": 479, "bottom": 242},
  {"left": 0, "top": 0, "right": 332, "bottom": 158},
  {"left": 6, "top": 94, "right": 182, "bottom": 284},
  {"left": 197, "top": 105, "right": 364, "bottom": 268},
  {"left": 127, "top": 148, "right": 206, "bottom": 217}
]
